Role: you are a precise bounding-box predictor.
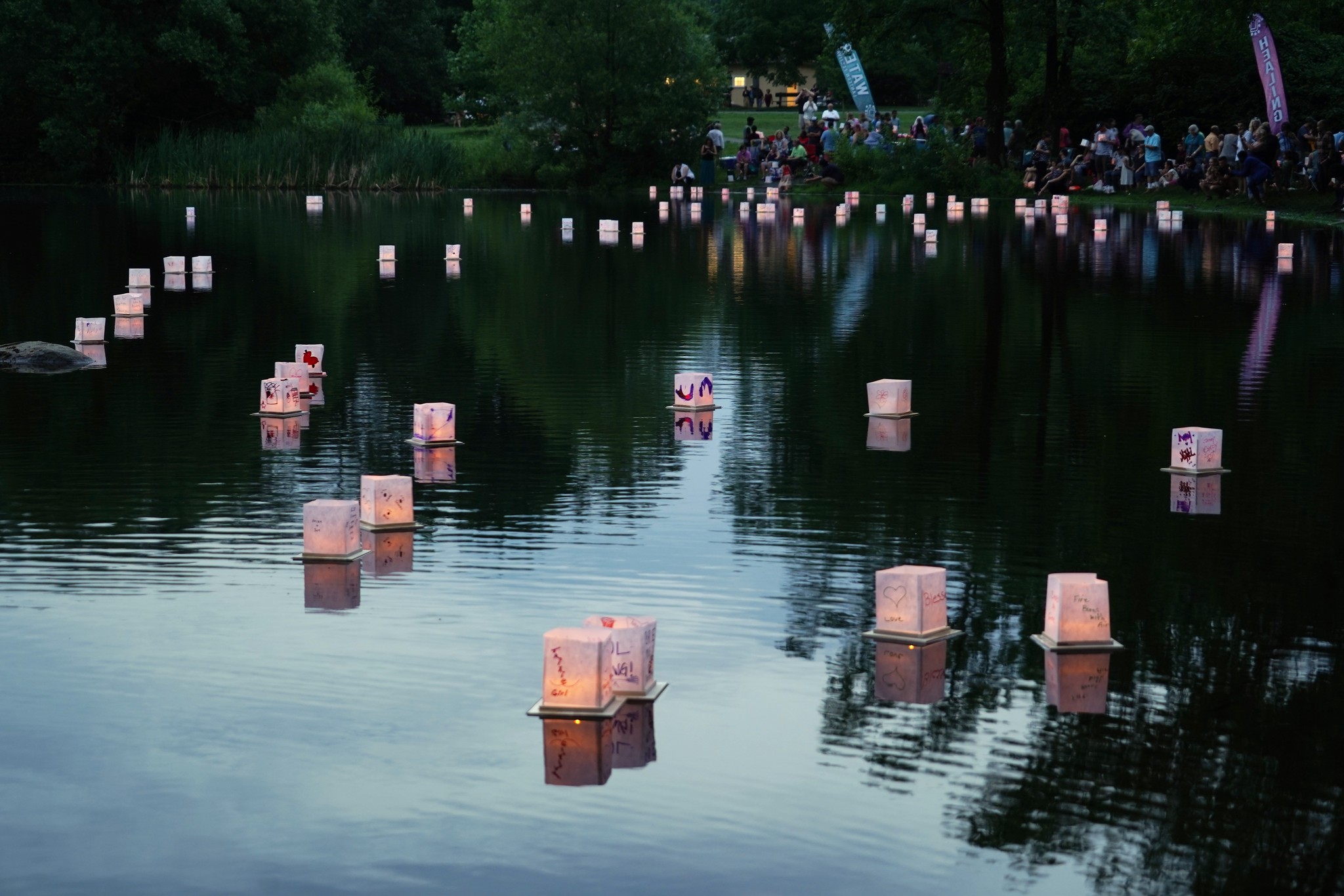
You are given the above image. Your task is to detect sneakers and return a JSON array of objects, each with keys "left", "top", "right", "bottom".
[
  {"left": 743, "top": 176, "right": 746, "bottom": 180},
  {"left": 737, "top": 176, "right": 740, "bottom": 180}
]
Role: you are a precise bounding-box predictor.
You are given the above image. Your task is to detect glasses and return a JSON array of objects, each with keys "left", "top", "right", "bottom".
[{"left": 1252, "top": 118, "right": 1258, "bottom": 119}]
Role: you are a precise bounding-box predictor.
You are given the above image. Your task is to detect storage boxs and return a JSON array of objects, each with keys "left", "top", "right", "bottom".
[{"left": 720, "top": 156, "right": 740, "bottom": 168}]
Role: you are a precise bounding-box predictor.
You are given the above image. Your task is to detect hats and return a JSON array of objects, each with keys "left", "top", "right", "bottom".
[
  {"left": 1143, "top": 125, "right": 1154, "bottom": 131},
  {"left": 1188, "top": 124, "right": 1198, "bottom": 133},
  {"left": 812, "top": 116, "right": 817, "bottom": 120},
  {"left": 1096, "top": 122, "right": 1104, "bottom": 130}
]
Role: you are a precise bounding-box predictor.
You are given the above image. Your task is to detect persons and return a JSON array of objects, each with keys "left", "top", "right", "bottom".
[
  {"left": 698, "top": 86, "right": 1344, "bottom": 215},
  {"left": 671, "top": 162, "right": 695, "bottom": 190}
]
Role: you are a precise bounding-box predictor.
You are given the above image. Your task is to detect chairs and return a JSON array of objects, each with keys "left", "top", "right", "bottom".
[{"left": 797, "top": 145, "right": 818, "bottom": 178}]
